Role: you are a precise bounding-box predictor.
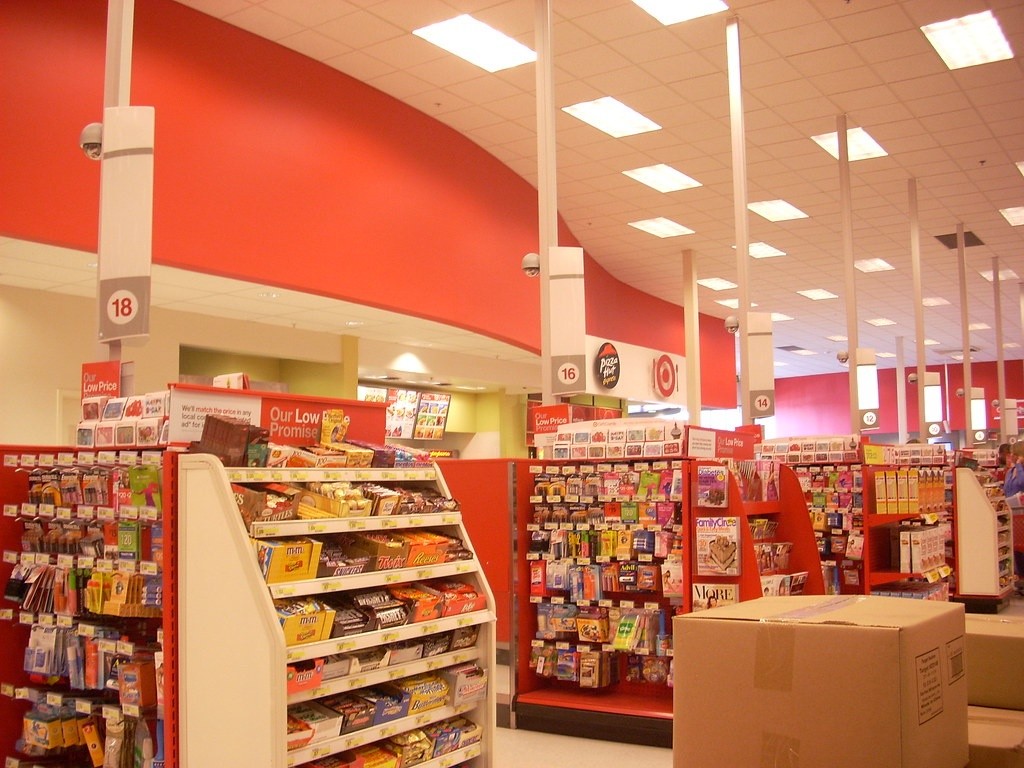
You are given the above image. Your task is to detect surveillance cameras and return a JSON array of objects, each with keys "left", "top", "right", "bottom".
[
  {"left": 725, "top": 317, "right": 740, "bottom": 334},
  {"left": 909, "top": 373, "right": 918, "bottom": 384},
  {"left": 837, "top": 351, "right": 849, "bottom": 363},
  {"left": 956, "top": 388, "right": 964, "bottom": 397},
  {"left": 522, "top": 253, "right": 540, "bottom": 278},
  {"left": 80, "top": 123, "right": 103, "bottom": 160},
  {"left": 992, "top": 400, "right": 999, "bottom": 407}
]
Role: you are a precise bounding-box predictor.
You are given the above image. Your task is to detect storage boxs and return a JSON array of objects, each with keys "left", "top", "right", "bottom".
[
  {"left": 695, "top": 517, "right": 742, "bottom": 577},
  {"left": 871, "top": 468, "right": 950, "bottom": 601},
  {"left": 230, "top": 479, "right": 487, "bottom": 768},
  {"left": 692, "top": 582, "right": 739, "bottom": 613},
  {"left": 672, "top": 595, "right": 970, "bottom": 768},
  {"left": 695, "top": 465, "right": 729, "bottom": 509},
  {"left": 964, "top": 612, "right": 1024, "bottom": 712},
  {"left": 966, "top": 703, "right": 1024, "bottom": 768}
]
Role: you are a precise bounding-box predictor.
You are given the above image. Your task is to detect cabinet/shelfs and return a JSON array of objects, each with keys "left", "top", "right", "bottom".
[
  {"left": 690, "top": 458, "right": 826, "bottom": 613},
  {"left": 177, "top": 452, "right": 498, "bottom": 768},
  {"left": 862, "top": 464, "right": 952, "bottom": 603},
  {"left": 956, "top": 467, "right": 1019, "bottom": 614}
]
